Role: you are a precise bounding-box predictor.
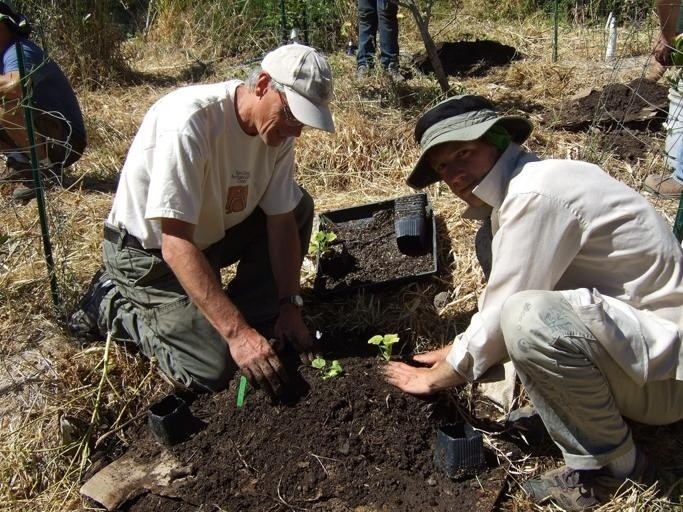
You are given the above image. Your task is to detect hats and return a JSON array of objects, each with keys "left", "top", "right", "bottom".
[
  {"left": 0, "top": 0, "right": 30, "bottom": 40},
  {"left": 259, "top": 44, "right": 335, "bottom": 134},
  {"left": 403, "top": 95, "right": 535, "bottom": 191}
]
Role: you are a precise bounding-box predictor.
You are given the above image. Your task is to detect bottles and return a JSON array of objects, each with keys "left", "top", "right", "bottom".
[{"left": 346, "top": 41, "right": 355, "bottom": 56}]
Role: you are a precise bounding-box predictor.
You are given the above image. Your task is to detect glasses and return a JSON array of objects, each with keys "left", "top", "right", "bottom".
[{"left": 277, "top": 87, "right": 303, "bottom": 128}]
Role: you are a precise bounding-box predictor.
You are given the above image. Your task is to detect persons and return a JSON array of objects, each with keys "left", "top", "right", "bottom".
[
  {"left": 377, "top": 93, "right": 683, "bottom": 512},
  {"left": 643, "top": 0, "right": 683, "bottom": 197},
  {"left": 352, "top": 0, "right": 412, "bottom": 82},
  {"left": 0, "top": 11, "right": 88, "bottom": 202},
  {"left": 64, "top": 43, "right": 336, "bottom": 398}
]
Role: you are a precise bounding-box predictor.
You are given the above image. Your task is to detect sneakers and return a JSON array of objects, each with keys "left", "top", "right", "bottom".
[
  {"left": 0, "top": 157, "right": 32, "bottom": 184},
  {"left": 640, "top": 171, "right": 683, "bottom": 200},
  {"left": 66, "top": 265, "right": 115, "bottom": 338},
  {"left": 101, "top": 227, "right": 160, "bottom": 255},
  {"left": 521, "top": 448, "right": 657, "bottom": 512},
  {"left": 355, "top": 61, "right": 406, "bottom": 83},
  {"left": 13, "top": 163, "right": 65, "bottom": 200}
]
